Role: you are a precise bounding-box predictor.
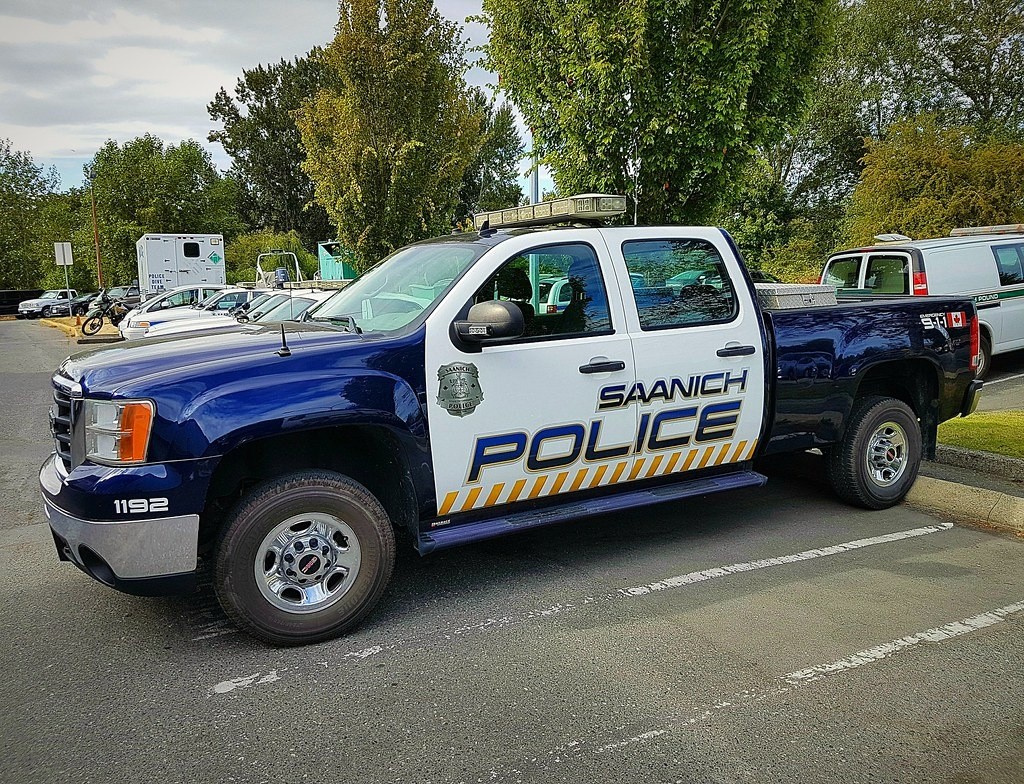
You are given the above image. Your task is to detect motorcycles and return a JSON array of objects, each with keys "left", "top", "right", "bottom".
[{"left": 81, "top": 294, "right": 134, "bottom": 336}]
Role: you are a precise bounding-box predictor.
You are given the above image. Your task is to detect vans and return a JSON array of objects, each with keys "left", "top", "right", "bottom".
[{"left": 816, "top": 223, "right": 1024, "bottom": 381}]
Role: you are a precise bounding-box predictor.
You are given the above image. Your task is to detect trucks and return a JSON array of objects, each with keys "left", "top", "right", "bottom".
[{"left": 135, "top": 233, "right": 225, "bottom": 311}]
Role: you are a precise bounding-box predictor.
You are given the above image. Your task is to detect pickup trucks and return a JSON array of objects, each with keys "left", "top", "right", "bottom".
[{"left": 36, "top": 192, "right": 982, "bottom": 648}]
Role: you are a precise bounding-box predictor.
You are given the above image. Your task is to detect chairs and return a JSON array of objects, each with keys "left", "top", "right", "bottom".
[
  {"left": 497, "top": 267, "right": 538, "bottom": 337},
  {"left": 556, "top": 258, "right": 608, "bottom": 332},
  {"left": 235, "top": 294, "right": 247, "bottom": 308},
  {"left": 680, "top": 283, "right": 727, "bottom": 322}
]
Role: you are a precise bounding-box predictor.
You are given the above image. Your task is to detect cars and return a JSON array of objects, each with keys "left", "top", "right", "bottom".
[{"left": 16, "top": 281, "right": 434, "bottom": 343}]
